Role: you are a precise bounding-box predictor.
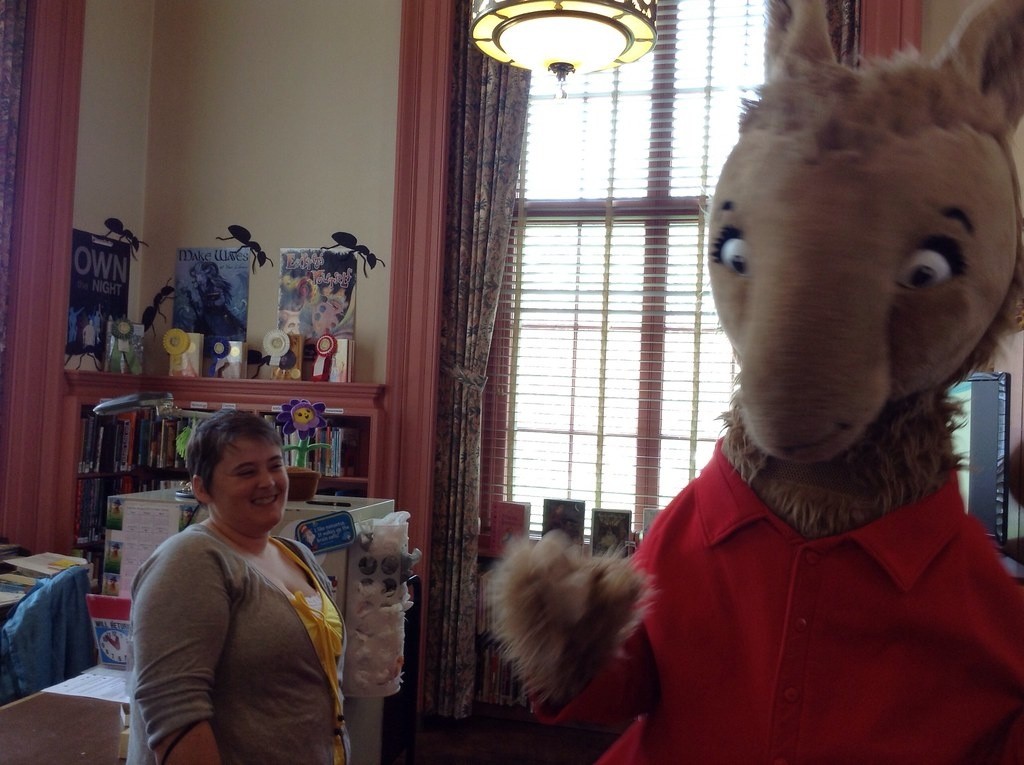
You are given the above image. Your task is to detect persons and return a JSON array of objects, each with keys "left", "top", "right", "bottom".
[{"left": 127, "top": 408, "right": 349, "bottom": 765}]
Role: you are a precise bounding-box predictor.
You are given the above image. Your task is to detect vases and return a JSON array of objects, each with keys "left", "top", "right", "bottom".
[{"left": 284, "top": 467, "right": 321, "bottom": 500}]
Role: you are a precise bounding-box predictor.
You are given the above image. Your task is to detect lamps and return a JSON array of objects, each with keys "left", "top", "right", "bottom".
[{"left": 467, "top": 0, "right": 658, "bottom": 84}]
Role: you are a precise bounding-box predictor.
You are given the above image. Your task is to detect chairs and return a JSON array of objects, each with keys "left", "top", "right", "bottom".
[{"left": 0, "top": 566, "right": 94, "bottom": 704}]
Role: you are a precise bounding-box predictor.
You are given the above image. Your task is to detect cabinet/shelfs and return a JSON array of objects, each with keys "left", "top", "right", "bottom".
[{"left": 58, "top": 370, "right": 389, "bottom": 594}]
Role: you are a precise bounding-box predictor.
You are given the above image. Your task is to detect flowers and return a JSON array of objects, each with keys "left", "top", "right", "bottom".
[{"left": 277, "top": 399, "right": 333, "bottom": 466}]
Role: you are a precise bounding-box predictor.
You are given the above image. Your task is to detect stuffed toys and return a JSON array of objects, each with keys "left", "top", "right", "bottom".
[{"left": 481, "top": 1, "right": 1024, "bottom": 765}]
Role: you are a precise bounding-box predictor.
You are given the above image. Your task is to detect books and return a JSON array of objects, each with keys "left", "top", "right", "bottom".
[
  {"left": 480, "top": 499, "right": 662, "bottom": 715},
  {"left": 72, "top": 321, "right": 357, "bottom": 598}
]
